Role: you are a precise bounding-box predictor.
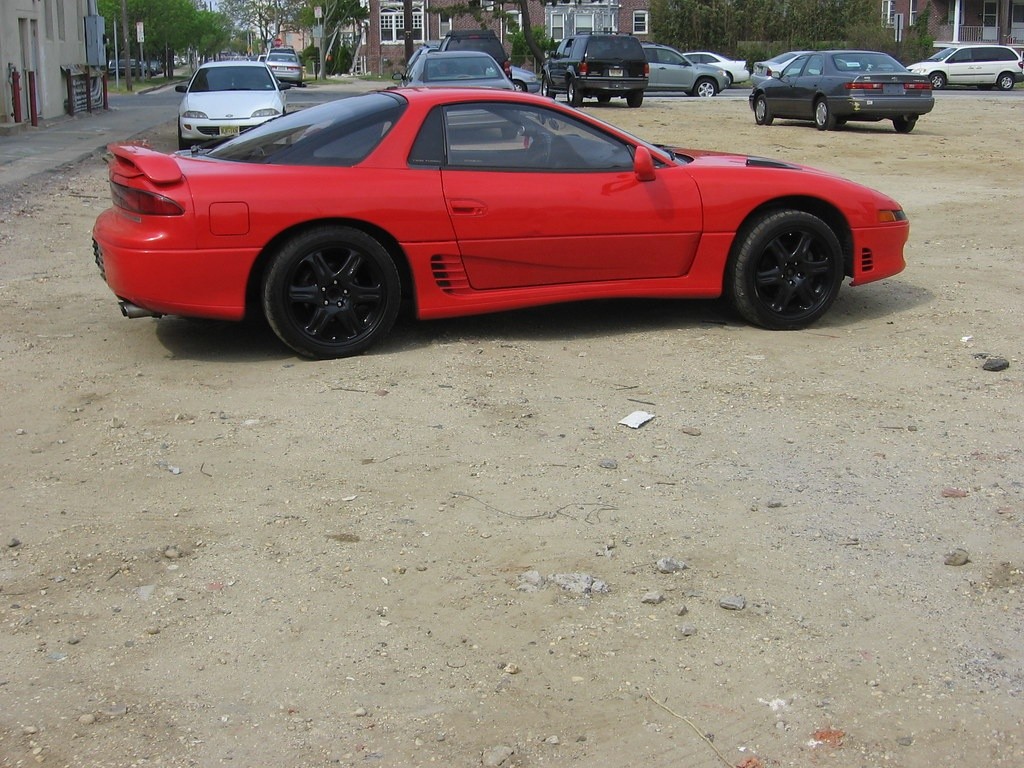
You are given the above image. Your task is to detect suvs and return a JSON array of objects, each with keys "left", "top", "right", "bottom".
[
  {"left": 640, "top": 40, "right": 732, "bottom": 98},
  {"left": 107, "top": 60, "right": 157, "bottom": 79},
  {"left": 541, "top": 28, "right": 651, "bottom": 109},
  {"left": 431, "top": 29, "right": 513, "bottom": 80}
]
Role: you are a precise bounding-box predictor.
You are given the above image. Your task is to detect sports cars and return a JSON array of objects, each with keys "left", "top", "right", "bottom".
[{"left": 91, "top": 83, "right": 913, "bottom": 361}]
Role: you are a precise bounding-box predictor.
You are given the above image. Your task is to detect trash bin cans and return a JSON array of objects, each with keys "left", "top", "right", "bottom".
[{"left": 312, "top": 60, "right": 320, "bottom": 74}]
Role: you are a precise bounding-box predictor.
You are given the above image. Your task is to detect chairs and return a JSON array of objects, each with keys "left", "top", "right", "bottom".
[{"left": 835, "top": 58, "right": 847, "bottom": 71}]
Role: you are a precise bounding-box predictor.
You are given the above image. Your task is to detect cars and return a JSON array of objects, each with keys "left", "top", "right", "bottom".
[
  {"left": 219, "top": 47, "right": 306, "bottom": 87},
  {"left": 175, "top": 60, "right": 290, "bottom": 150},
  {"left": 666, "top": 51, "right": 751, "bottom": 88},
  {"left": 747, "top": 49, "right": 936, "bottom": 134},
  {"left": 404, "top": 43, "right": 542, "bottom": 94},
  {"left": 391, "top": 49, "right": 519, "bottom": 140},
  {"left": 750, "top": 50, "right": 873, "bottom": 87},
  {"left": 904, "top": 43, "right": 1024, "bottom": 92}
]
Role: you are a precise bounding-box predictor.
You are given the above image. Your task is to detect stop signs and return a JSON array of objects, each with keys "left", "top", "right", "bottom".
[{"left": 273, "top": 38, "right": 282, "bottom": 48}]
